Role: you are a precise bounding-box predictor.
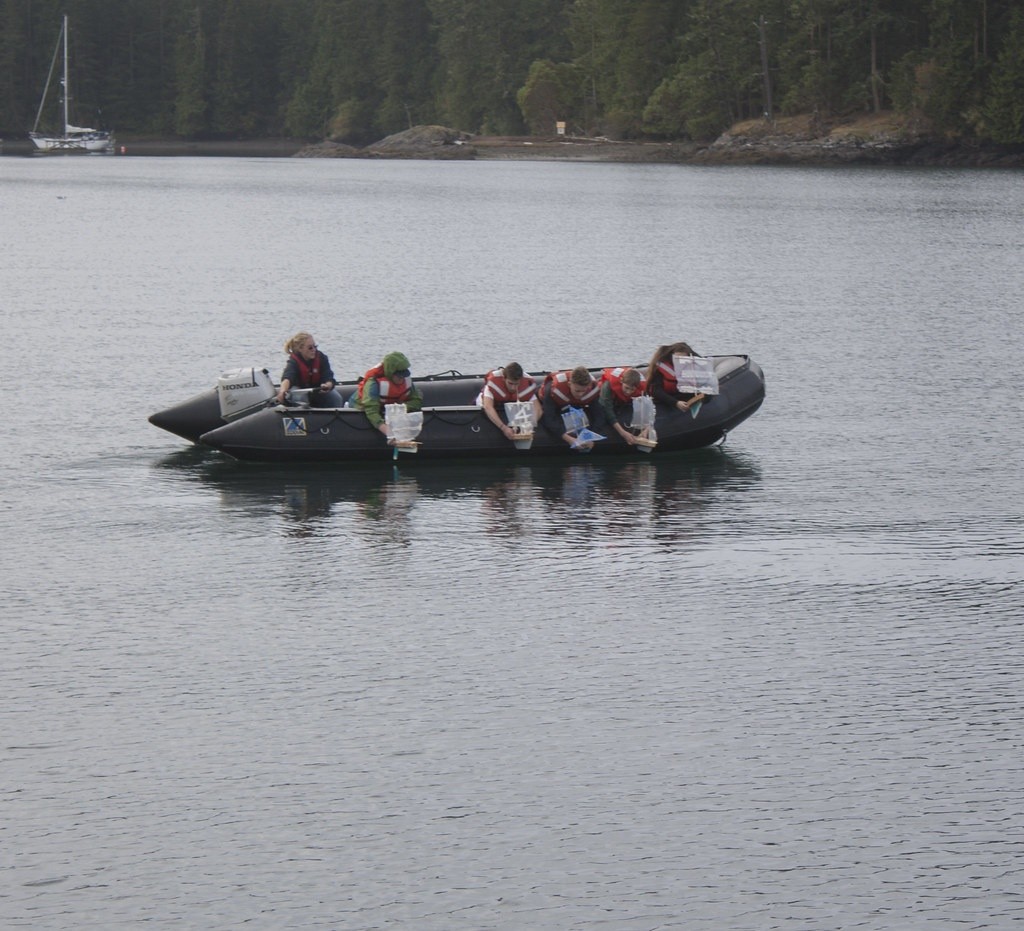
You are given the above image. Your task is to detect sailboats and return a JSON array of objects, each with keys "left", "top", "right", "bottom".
[{"left": 27, "top": 14, "right": 119, "bottom": 154}]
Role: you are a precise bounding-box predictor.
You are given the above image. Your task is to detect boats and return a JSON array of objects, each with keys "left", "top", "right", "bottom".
[{"left": 146, "top": 353, "right": 767, "bottom": 466}]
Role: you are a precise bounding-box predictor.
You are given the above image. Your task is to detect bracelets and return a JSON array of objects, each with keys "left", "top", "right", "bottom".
[{"left": 499, "top": 423, "right": 504, "bottom": 429}]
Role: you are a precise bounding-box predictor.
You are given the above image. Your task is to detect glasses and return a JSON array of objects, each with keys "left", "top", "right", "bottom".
[{"left": 309, "top": 345, "right": 317, "bottom": 350}]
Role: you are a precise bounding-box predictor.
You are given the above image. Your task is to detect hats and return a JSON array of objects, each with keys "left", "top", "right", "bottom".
[{"left": 395, "top": 368, "right": 410, "bottom": 376}]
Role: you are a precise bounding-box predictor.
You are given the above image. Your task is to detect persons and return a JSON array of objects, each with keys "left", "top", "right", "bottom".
[
  {"left": 598, "top": 367, "right": 647, "bottom": 446},
  {"left": 646, "top": 343, "right": 713, "bottom": 412},
  {"left": 471, "top": 362, "right": 542, "bottom": 441},
  {"left": 348, "top": 351, "right": 423, "bottom": 437},
  {"left": 276, "top": 332, "right": 343, "bottom": 408},
  {"left": 535, "top": 366, "right": 599, "bottom": 449}
]
